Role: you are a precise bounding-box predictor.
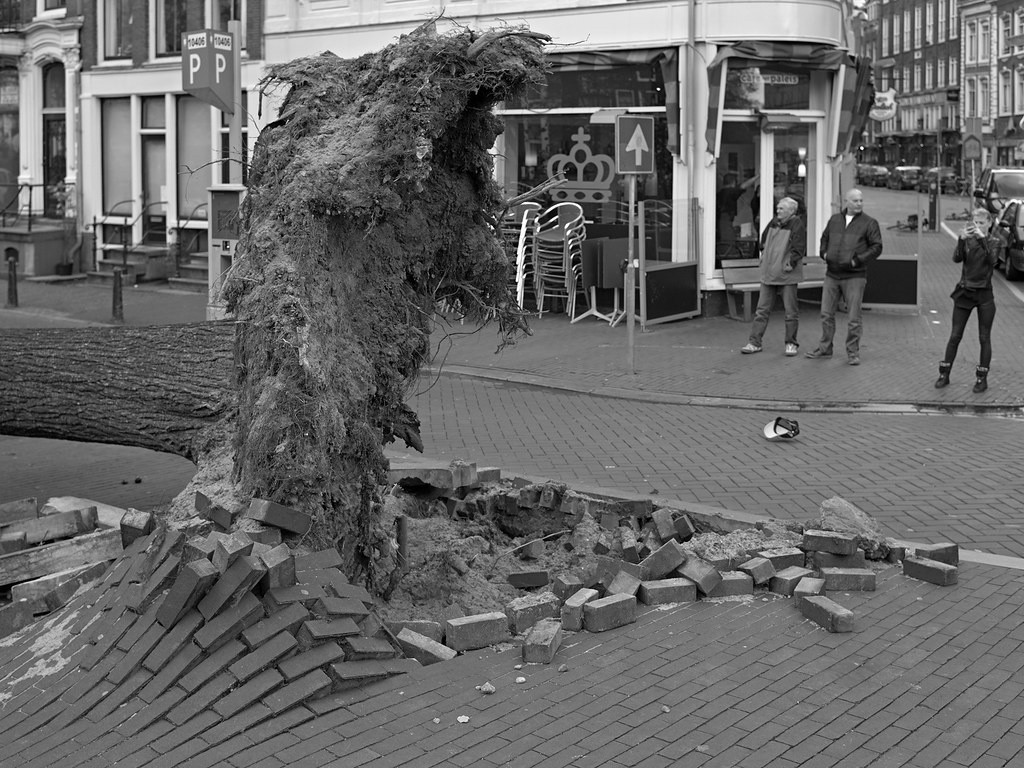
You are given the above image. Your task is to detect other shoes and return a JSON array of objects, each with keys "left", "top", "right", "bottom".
[
  {"left": 741, "top": 343, "right": 762, "bottom": 353},
  {"left": 804, "top": 348, "right": 832, "bottom": 359},
  {"left": 785, "top": 343, "right": 797, "bottom": 355},
  {"left": 848, "top": 356, "right": 860, "bottom": 365}
]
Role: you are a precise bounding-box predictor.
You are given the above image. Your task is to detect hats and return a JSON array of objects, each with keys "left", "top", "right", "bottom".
[{"left": 764, "top": 416, "right": 800, "bottom": 438}]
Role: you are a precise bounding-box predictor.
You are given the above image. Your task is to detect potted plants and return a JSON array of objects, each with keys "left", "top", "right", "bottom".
[{"left": 46, "top": 181, "right": 81, "bottom": 274}]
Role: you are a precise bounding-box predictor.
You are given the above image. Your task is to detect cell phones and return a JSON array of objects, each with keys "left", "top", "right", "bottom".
[{"left": 966, "top": 223, "right": 975, "bottom": 235}]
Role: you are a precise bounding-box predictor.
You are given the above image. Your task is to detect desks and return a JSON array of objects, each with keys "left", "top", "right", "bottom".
[{"left": 568, "top": 236, "right": 641, "bottom": 326}]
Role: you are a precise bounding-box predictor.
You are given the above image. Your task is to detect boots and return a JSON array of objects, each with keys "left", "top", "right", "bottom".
[
  {"left": 973, "top": 365, "right": 990, "bottom": 393},
  {"left": 935, "top": 361, "right": 953, "bottom": 388}
]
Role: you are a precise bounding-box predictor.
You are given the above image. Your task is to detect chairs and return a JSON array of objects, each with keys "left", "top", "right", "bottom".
[{"left": 442, "top": 200, "right": 587, "bottom": 319}]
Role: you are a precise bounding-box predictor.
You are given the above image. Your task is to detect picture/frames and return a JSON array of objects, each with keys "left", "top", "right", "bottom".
[
  {"left": 639, "top": 90, "right": 659, "bottom": 114},
  {"left": 637, "top": 67, "right": 656, "bottom": 82},
  {"left": 615, "top": 89, "right": 635, "bottom": 107}
]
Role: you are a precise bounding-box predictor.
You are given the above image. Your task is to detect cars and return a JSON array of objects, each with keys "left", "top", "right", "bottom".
[
  {"left": 857, "top": 163, "right": 960, "bottom": 195},
  {"left": 990, "top": 198, "right": 1024, "bottom": 281}
]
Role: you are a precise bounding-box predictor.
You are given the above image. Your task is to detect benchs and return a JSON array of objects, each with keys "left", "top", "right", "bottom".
[{"left": 721, "top": 255, "right": 848, "bottom": 323}]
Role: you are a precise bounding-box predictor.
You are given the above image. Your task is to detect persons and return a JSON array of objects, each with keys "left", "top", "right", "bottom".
[
  {"left": 717, "top": 172, "right": 760, "bottom": 230},
  {"left": 935, "top": 208, "right": 1001, "bottom": 392},
  {"left": 804, "top": 189, "right": 883, "bottom": 365},
  {"left": 740, "top": 197, "right": 806, "bottom": 356}
]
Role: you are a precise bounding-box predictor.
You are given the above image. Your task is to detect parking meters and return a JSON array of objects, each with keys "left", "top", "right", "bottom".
[{"left": 206, "top": 184, "right": 248, "bottom": 322}]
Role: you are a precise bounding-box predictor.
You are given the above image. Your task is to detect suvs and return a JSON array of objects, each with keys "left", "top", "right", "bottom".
[{"left": 974, "top": 165, "right": 1024, "bottom": 233}]
